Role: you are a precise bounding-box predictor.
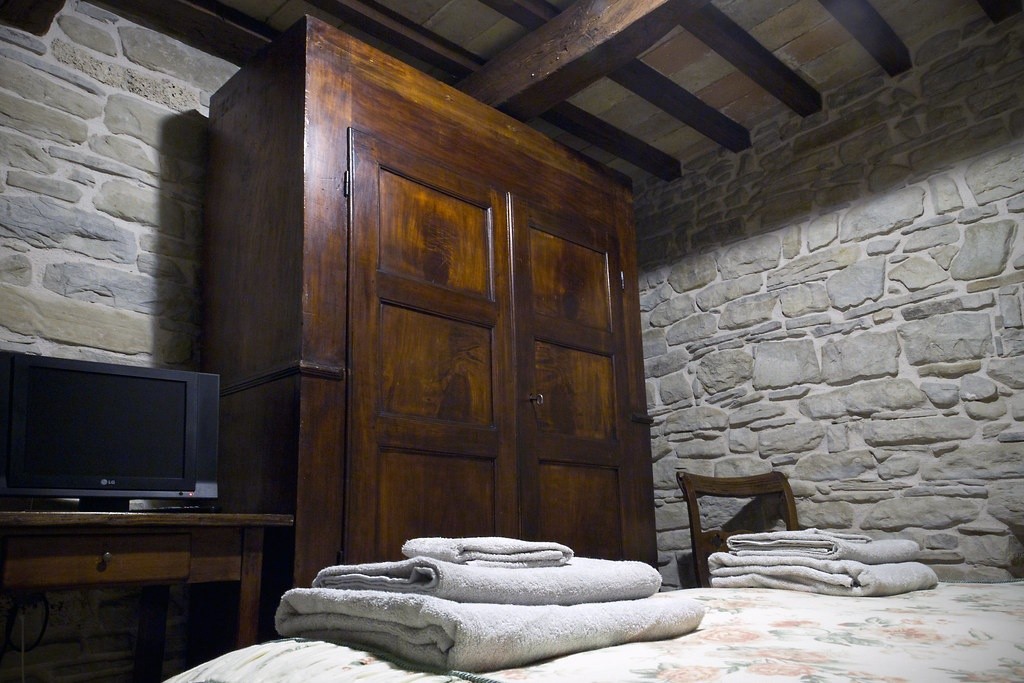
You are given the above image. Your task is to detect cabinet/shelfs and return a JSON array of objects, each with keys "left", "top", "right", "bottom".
[{"left": 208, "top": 16, "right": 658, "bottom": 646}]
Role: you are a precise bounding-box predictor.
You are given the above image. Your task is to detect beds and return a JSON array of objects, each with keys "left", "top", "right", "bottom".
[{"left": 170, "top": 575, "right": 1024, "bottom": 683}]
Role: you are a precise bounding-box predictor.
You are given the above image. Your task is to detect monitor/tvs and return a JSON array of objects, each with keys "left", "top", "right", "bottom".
[{"left": 0, "top": 349, "right": 219, "bottom": 511}]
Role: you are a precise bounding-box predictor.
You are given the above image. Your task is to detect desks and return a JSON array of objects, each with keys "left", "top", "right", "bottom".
[{"left": 0, "top": 508, "right": 295, "bottom": 683}]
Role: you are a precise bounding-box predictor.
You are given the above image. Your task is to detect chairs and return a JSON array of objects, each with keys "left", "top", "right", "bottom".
[{"left": 677, "top": 470, "right": 799, "bottom": 588}]
